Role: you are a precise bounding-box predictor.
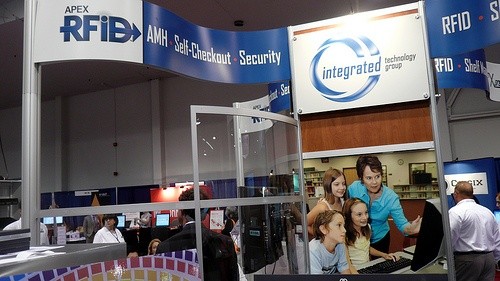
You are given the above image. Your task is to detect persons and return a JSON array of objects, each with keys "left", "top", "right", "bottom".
[
  {"left": 3, "top": 200, "right": 50, "bottom": 247},
  {"left": 82, "top": 215, "right": 102, "bottom": 243},
  {"left": 135, "top": 211, "right": 153, "bottom": 230},
  {"left": 169, "top": 220, "right": 181, "bottom": 229},
  {"left": 346, "top": 155, "right": 422, "bottom": 261},
  {"left": 148, "top": 239, "right": 161, "bottom": 256},
  {"left": 156, "top": 188, "right": 240, "bottom": 281},
  {"left": 309, "top": 209, "right": 351, "bottom": 275},
  {"left": 448, "top": 181, "right": 500, "bottom": 281},
  {"left": 307, "top": 169, "right": 348, "bottom": 241},
  {"left": 93, "top": 214, "right": 125, "bottom": 244},
  {"left": 289, "top": 193, "right": 311, "bottom": 240},
  {"left": 342, "top": 197, "right": 401, "bottom": 275},
  {"left": 222, "top": 206, "right": 254, "bottom": 281}
]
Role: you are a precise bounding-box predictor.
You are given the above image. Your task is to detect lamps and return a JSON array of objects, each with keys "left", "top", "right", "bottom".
[{"left": 270, "top": 169, "right": 274, "bottom": 176}]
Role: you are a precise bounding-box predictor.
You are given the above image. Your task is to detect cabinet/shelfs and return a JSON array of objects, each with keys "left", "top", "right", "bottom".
[
  {"left": 0, "top": 179, "right": 23, "bottom": 206},
  {"left": 304, "top": 170, "right": 327, "bottom": 197},
  {"left": 393, "top": 183, "right": 440, "bottom": 199}
]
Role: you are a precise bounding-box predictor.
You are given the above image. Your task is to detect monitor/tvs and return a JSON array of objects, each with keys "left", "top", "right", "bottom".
[
  {"left": 155, "top": 213, "right": 170, "bottom": 227},
  {"left": 238, "top": 186, "right": 285, "bottom": 275},
  {"left": 116, "top": 215, "right": 126, "bottom": 228},
  {"left": 411, "top": 198, "right": 445, "bottom": 272},
  {"left": 43, "top": 216, "right": 63, "bottom": 224}
]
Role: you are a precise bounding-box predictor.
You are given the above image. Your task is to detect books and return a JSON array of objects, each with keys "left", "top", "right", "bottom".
[{"left": 0, "top": 245, "right": 66, "bottom": 267}]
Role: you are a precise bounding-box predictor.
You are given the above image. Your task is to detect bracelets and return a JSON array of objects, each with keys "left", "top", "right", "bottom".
[{"left": 291, "top": 204, "right": 295, "bottom": 212}]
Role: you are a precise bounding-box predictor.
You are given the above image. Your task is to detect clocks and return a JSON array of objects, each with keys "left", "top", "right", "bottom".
[{"left": 398, "top": 159, "right": 405, "bottom": 166}]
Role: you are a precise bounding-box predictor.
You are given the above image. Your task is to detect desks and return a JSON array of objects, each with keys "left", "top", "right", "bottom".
[
  {"left": 402, "top": 244, "right": 447, "bottom": 265},
  {"left": 355, "top": 251, "right": 448, "bottom": 274}
]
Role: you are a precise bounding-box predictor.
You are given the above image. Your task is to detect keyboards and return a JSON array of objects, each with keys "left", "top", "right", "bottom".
[{"left": 356, "top": 255, "right": 413, "bottom": 274}]
